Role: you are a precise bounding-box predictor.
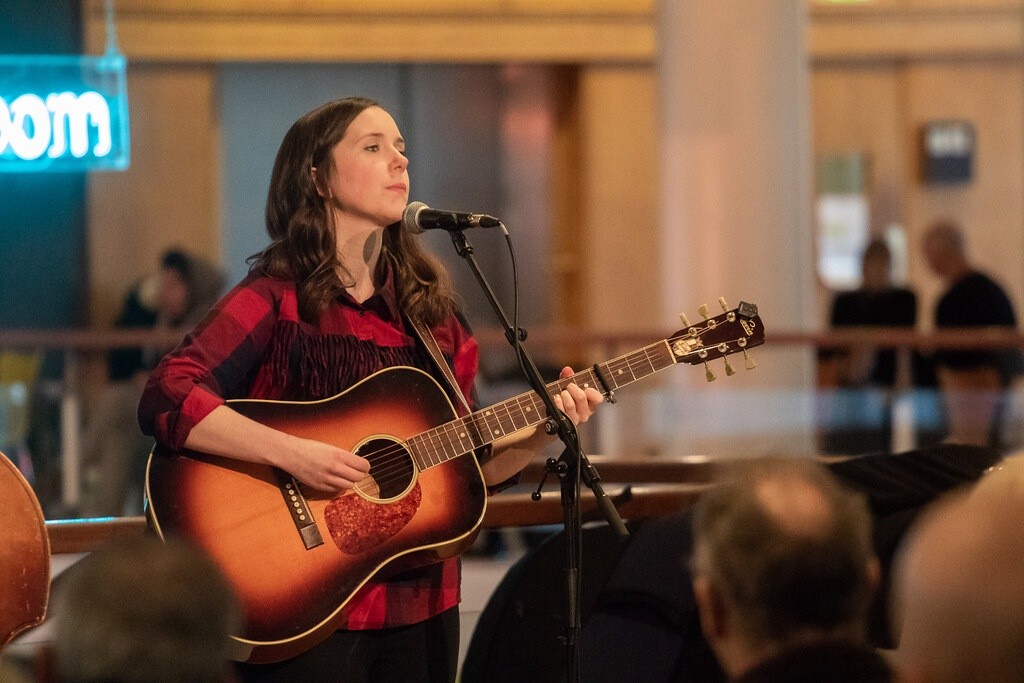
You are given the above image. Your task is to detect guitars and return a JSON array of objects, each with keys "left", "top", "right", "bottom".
[{"left": 141, "top": 293, "right": 770, "bottom": 669}]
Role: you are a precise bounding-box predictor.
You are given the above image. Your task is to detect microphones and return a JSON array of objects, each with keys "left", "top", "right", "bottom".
[{"left": 401, "top": 201, "right": 499, "bottom": 235}]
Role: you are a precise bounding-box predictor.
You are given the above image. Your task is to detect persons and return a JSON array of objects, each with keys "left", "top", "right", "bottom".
[
  {"left": 136, "top": 95, "right": 603, "bottom": 683},
  {"left": 103, "top": 248, "right": 195, "bottom": 521},
  {"left": 827, "top": 219, "right": 1023, "bottom": 454},
  {"left": 688, "top": 453, "right": 1024, "bottom": 683}
]
